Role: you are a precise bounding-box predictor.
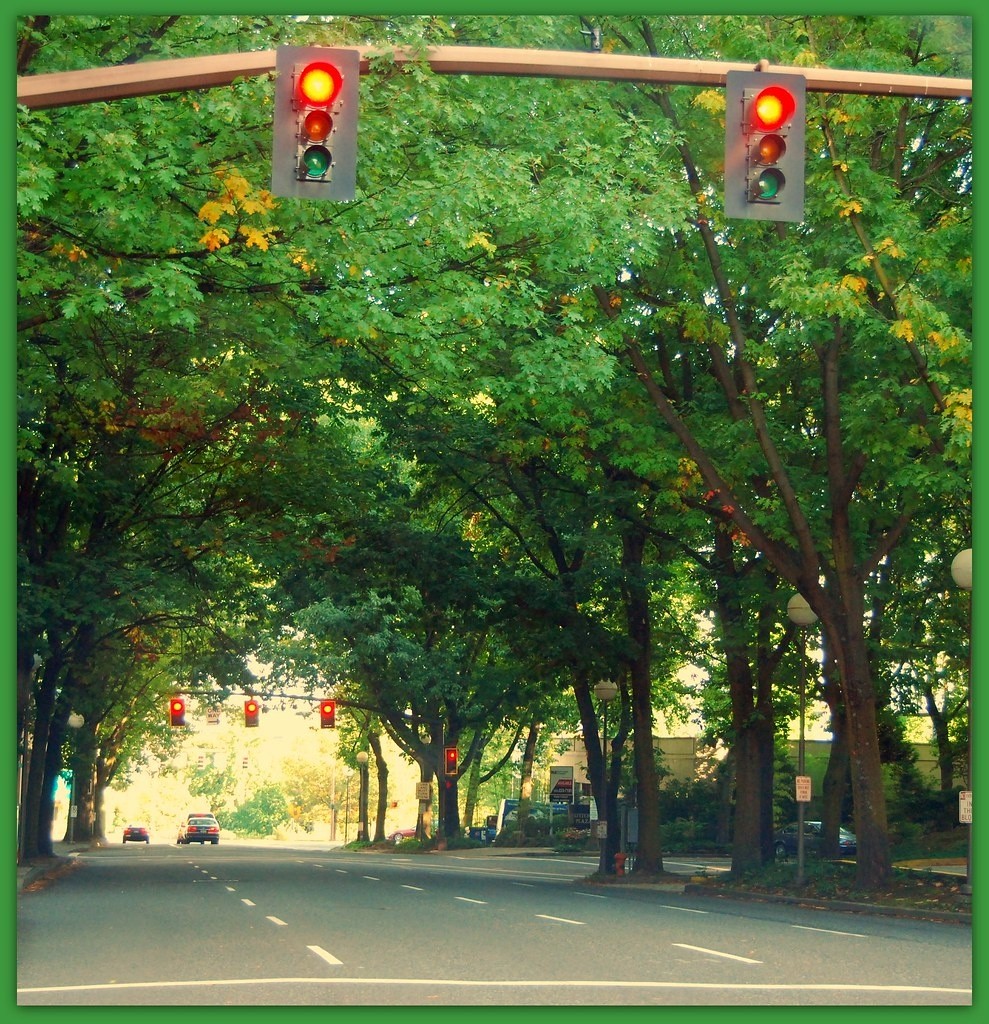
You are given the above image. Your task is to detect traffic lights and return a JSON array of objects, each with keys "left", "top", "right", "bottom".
[
  {"left": 245, "top": 700, "right": 259, "bottom": 727},
  {"left": 171, "top": 699, "right": 185, "bottom": 726},
  {"left": 445, "top": 748, "right": 458, "bottom": 775},
  {"left": 270, "top": 45, "right": 358, "bottom": 203},
  {"left": 320, "top": 700, "right": 335, "bottom": 728},
  {"left": 723, "top": 65, "right": 805, "bottom": 223}
]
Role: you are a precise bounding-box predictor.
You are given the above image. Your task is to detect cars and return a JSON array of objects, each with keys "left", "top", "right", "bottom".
[
  {"left": 388, "top": 819, "right": 438, "bottom": 840},
  {"left": 773, "top": 821, "right": 857, "bottom": 858},
  {"left": 123, "top": 825, "right": 149, "bottom": 844},
  {"left": 177, "top": 812, "right": 219, "bottom": 844}
]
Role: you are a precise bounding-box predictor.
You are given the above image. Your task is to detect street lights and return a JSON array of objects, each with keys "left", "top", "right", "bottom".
[
  {"left": 787, "top": 594, "right": 820, "bottom": 883},
  {"left": 65, "top": 712, "right": 84, "bottom": 845},
  {"left": 596, "top": 680, "right": 619, "bottom": 876},
  {"left": 951, "top": 547, "right": 975, "bottom": 893},
  {"left": 356, "top": 751, "right": 369, "bottom": 844}
]
